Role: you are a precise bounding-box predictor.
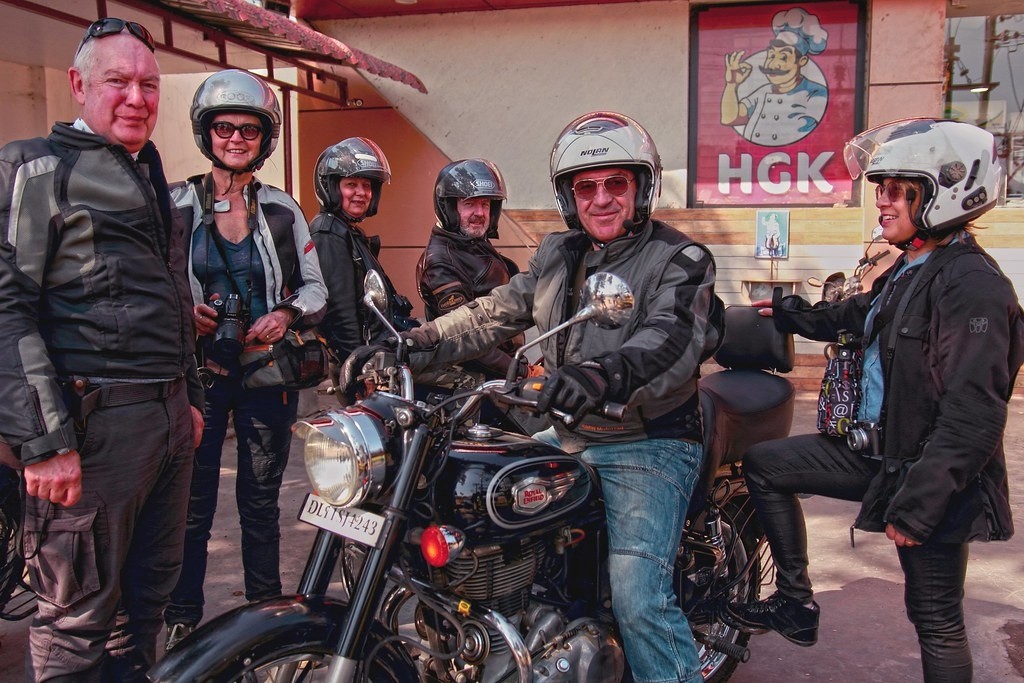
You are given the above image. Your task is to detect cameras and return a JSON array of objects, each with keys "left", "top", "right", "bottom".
[
  {"left": 847, "top": 420, "right": 884, "bottom": 460},
  {"left": 205, "top": 294, "right": 252, "bottom": 358}
]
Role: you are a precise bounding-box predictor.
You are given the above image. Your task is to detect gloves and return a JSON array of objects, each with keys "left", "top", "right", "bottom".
[
  {"left": 340, "top": 336, "right": 400, "bottom": 398},
  {"left": 536, "top": 358, "right": 612, "bottom": 430}
]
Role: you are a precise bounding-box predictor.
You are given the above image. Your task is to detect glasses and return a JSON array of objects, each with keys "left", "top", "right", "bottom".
[
  {"left": 76, "top": 18, "right": 155, "bottom": 66},
  {"left": 571, "top": 174, "right": 638, "bottom": 201},
  {"left": 210, "top": 121, "right": 268, "bottom": 141},
  {"left": 875, "top": 181, "right": 923, "bottom": 202}
]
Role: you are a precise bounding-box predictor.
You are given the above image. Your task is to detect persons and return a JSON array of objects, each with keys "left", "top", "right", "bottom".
[
  {"left": 164, "top": 67, "right": 330, "bottom": 653},
  {"left": 0, "top": 17, "right": 205, "bottom": 683},
  {"left": 718, "top": 117, "right": 1023, "bottom": 683},
  {"left": 339, "top": 111, "right": 725, "bottom": 683},
  {"left": 308, "top": 139, "right": 441, "bottom": 374},
  {"left": 415, "top": 159, "right": 544, "bottom": 382}
]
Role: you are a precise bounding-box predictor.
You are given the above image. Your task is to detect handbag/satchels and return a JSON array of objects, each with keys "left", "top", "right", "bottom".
[
  {"left": 231, "top": 327, "right": 330, "bottom": 389},
  {"left": 817, "top": 330, "right": 868, "bottom": 438}
]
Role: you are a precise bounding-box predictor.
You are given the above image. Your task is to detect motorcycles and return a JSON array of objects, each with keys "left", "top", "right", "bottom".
[{"left": 188, "top": 298, "right": 797, "bottom": 683}]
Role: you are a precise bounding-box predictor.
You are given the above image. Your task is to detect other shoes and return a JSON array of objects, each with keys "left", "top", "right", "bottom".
[
  {"left": 163, "top": 623, "right": 195, "bottom": 654},
  {"left": 298, "top": 659, "right": 323, "bottom": 669}
]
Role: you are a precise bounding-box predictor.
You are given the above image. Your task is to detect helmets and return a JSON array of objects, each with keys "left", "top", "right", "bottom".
[
  {"left": 189, "top": 69, "right": 282, "bottom": 173},
  {"left": 864, "top": 119, "right": 1002, "bottom": 231},
  {"left": 550, "top": 110, "right": 662, "bottom": 232},
  {"left": 433, "top": 159, "right": 508, "bottom": 236},
  {"left": 312, "top": 144, "right": 392, "bottom": 217}
]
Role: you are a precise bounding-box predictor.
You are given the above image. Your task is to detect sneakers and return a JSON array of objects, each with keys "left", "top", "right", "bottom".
[{"left": 725, "top": 590, "right": 820, "bottom": 647}]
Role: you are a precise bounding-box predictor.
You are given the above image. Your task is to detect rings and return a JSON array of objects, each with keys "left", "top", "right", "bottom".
[
  {"left": 267, "top": 335, "right": 270, "bottom": 339},
  {"left": 906, "top": 540, "right": 912, "bottom": 544},
  {"left": 197, "top": 316, "right": 202, "bottom": 322}
]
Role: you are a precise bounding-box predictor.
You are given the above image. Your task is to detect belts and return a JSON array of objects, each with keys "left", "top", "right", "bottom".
[{"left": 73, "top": 374, "right": 184, "bottom": 419}]
